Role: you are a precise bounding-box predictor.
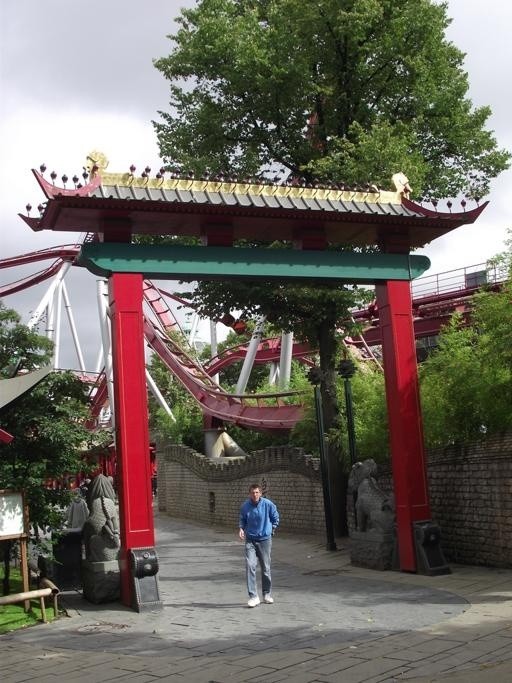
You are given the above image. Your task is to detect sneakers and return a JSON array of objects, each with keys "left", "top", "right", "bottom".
[
  {"left": 263, "top": 594, "right": 274, "bottom": 604},
  {"left": 247, "top": 594, "right": 261, "bottom": 608}
]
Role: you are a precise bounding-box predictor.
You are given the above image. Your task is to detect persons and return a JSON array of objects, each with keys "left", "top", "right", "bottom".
[{"left": 238, "top": 483, "right": 280, "bottom": 608}]
